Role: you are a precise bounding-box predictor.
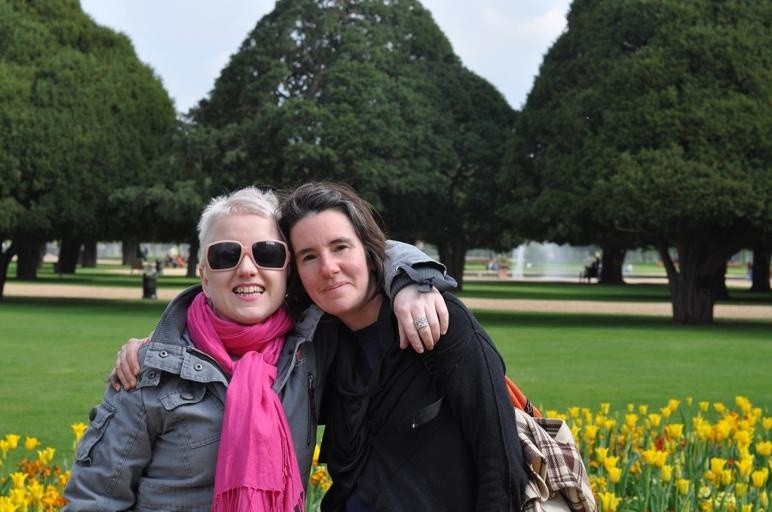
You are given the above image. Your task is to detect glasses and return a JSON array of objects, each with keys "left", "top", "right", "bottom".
[{"left": 205, "top": 239, "right": 291, "bottom": 272}]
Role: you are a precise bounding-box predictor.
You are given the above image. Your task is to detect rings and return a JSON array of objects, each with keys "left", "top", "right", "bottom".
[{"left": 413, "top": 318, "right": 429, "bottom": 331}]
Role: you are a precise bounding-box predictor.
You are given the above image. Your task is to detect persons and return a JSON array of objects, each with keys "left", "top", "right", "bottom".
[
  {"left": 108, "top": 180, "right": 528, "bottom": 512},
  {"left": 59, "top": 186, "right": 458, "bottom": 512}
]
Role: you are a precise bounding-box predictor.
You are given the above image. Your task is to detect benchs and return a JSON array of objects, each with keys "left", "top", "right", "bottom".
[{"left": 579, "top": 274, "right": 599, "bottom": 283}]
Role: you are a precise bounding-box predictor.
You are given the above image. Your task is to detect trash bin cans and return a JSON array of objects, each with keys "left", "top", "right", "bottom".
[{"left": 143, "top": 272, "right": 157, "bottom": 298}]
